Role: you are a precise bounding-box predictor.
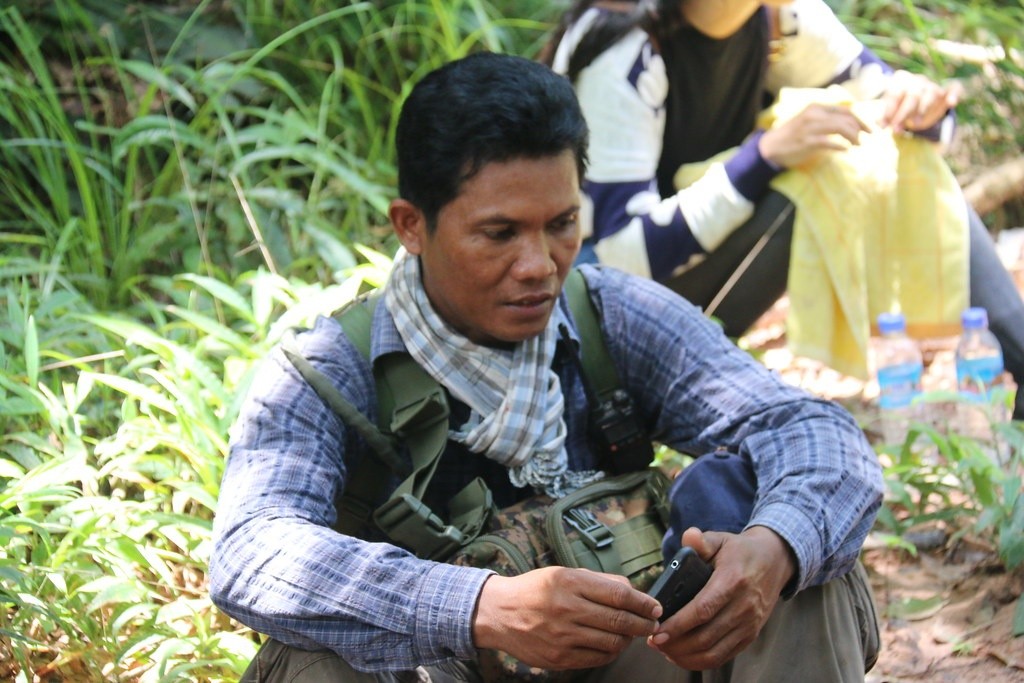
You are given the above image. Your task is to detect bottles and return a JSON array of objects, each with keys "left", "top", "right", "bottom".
[
  {"left": 871, "top": 312, "right": 939, "bottom": 468},
  {"left": 954, "top": 306, "right": 1011, "bottom": 465}
]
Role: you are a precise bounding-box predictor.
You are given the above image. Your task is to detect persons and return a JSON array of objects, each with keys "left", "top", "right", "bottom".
[
  {"left": 536, "top": 0, "right": 1024, "bottom": 422},
  {"left": 208, "top": 51, "right": 884, "bottom": 683}
]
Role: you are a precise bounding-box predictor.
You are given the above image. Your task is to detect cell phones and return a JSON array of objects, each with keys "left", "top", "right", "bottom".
[{"left": 645, "top": 545, "right": 713, "bottom": 624}]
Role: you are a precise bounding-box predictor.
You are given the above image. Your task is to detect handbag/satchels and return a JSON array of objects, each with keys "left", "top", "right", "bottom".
[{"left": 438, "top": 467, "right": 678, "bottom": 683}]
